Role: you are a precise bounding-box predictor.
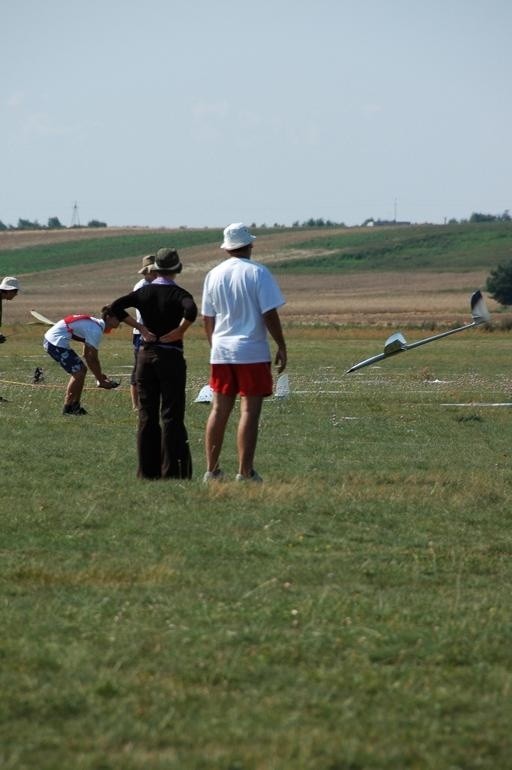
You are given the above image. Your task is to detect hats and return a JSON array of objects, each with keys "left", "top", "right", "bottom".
[
  {"left": 220, "top": 223, "right": 256, "bottom": 250},
  {"left": 137, "top": 248, "right": 182, "bottom": 274},
  {"left": 0, "top": 277, "right": 19, "bottom": 290}
]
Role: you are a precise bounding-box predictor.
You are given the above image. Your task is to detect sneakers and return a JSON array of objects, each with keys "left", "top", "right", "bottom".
[
  {"left": 62, "top": 407, "right": 92, "bottom": 417},
  {"left": 235, "top": 470, "right": 263, "bottom": 483},
  {"left": 203, "top": 470, "right": 230, "bottom": 483}
]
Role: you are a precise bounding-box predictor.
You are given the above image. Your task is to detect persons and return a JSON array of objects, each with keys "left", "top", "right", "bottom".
[
  {"left": 0, "top": 276, "right": 21, "bottom": 403},
  {"left": 41, "top": 303, "right": 119, "bottom": 416},
  {"left": 131, "top": 255, "right": 157, "bottom": 411},
  {"left": 199, "top": 221, "right": 287, "bottom": 485},
  {"left": 109, "top": 248, "right": 199, "bottom": 481}
]
